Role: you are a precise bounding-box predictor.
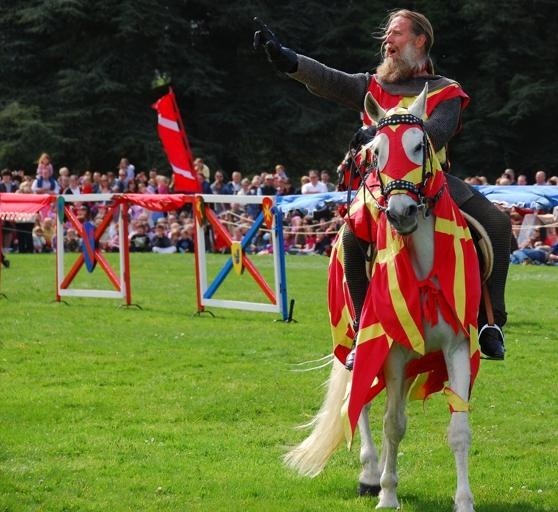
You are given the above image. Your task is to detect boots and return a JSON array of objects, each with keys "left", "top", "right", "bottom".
[
  {"left": 345, "top": 318, "right": 360, "bottom": 371},
  {"left": 477, "top": 316, "right": 505, "bottom": 361}
]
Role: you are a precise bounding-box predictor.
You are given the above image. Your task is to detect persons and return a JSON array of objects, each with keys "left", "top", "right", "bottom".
[
  {"left": 249, "top": 9, "right": 514, "bottom": 372},
  {"left": 465, "top": 169, "right": 558, "bottom": 266},
  {"left": 0, "top": 152, "right": 348, "bottom": 256}
]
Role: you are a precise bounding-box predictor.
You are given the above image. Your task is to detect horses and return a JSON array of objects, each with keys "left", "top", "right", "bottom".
[{"left": 278, "top": 82, "right": 476, "bottom": 512}]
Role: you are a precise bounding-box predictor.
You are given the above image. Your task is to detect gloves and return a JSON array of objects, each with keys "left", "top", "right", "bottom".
[
  {"left": 349, "top": 125, "right": 378, "bottom": 153},
  {"left": 250, "top": 16, "right": 297, "bottom": 74}
]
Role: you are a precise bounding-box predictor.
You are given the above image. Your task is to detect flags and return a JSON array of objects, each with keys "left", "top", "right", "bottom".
[{"left": 154, "top": 87, "right": 202, "bottom": 195}]
